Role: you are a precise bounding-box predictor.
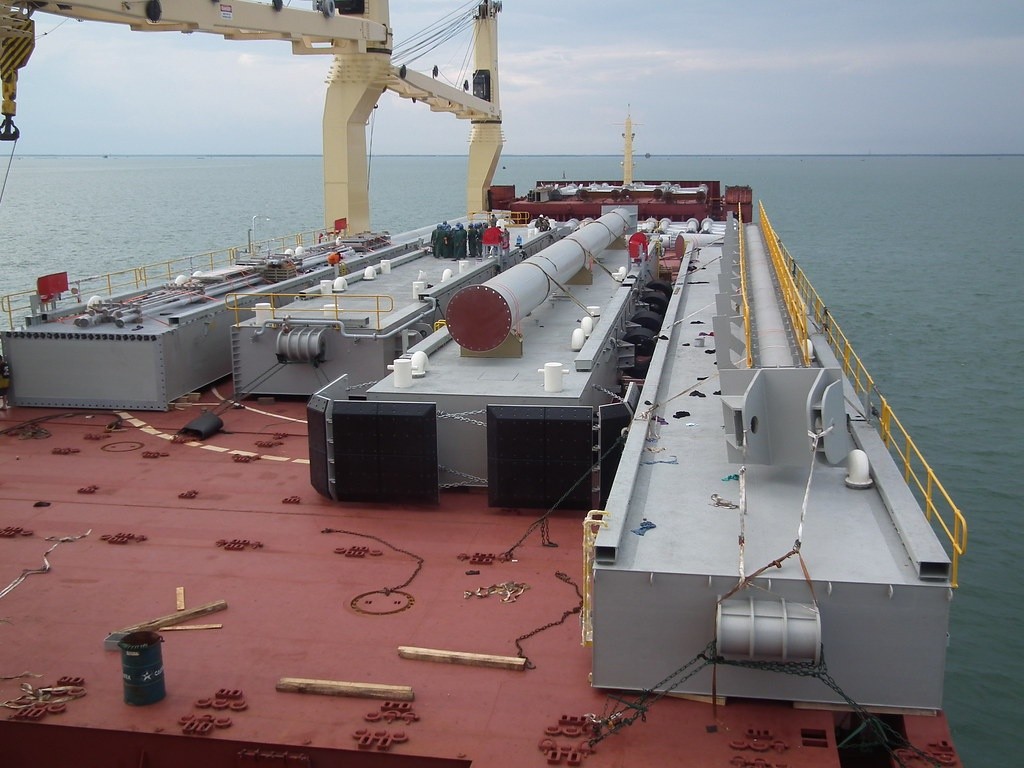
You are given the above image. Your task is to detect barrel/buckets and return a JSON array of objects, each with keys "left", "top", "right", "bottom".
[{"left": 117, "top": 631, "right": 166, "bottom": 706}]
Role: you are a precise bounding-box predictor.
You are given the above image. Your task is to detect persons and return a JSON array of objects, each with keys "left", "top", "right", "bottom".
[
  {"left": 535, "top": 215, "right": 551, "bottom": 231},
  {"left": 489, "top": 214, "right": 498, "bottom": 227},
  {"left": 431, "top": 221, "right": 489, "bottom": 260}
]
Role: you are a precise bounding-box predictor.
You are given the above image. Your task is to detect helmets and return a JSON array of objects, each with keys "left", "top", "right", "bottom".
[
  {"left": 539, "top": 214, "right": 543, "bottom": 218},
  {"left": 443, "top": 221, "right": 447, "bottom": 225},
  {"left": 437, "top": 224, "right": 442, "bottom": 229},
  {"left": 447, "top": 225, "right": 451, "bottom": 228},
  {"left": 545, "top": 216, "right": 549, "bottom": 220},
  {"left": 459, "top": 225, "right": 463, "bottom": 229},
  {"left": 491, "top": 213, "right": 495, "bottom": 216},
  {"left": 456, "top": 224, "right": 459, "bottom": 226},
  {"left": 469, "top": 222, "right": 488, "bottom": 228}
]
